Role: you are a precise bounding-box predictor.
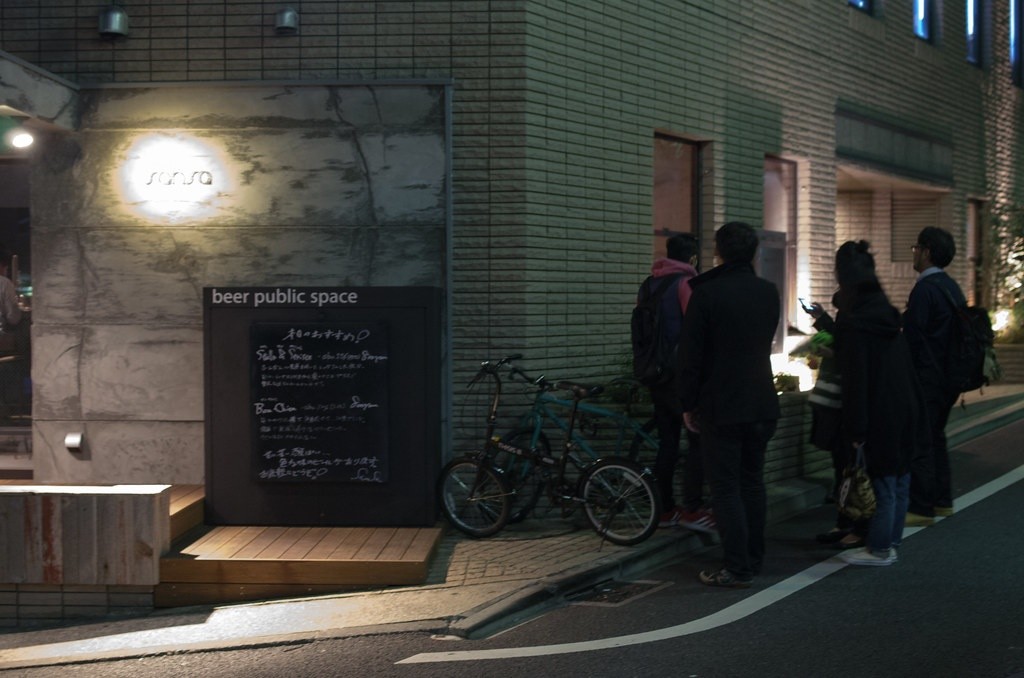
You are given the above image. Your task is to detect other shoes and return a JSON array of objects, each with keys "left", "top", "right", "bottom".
[
  {"left": 933, "top": 506, "right": 955, "bottom": 520},
  {"left": 815, "top": 527, "right": 865, "bottom": 549},
  {"left": 701, "top": 559, "right": 756, "bottom": 588},
  {"left": 840, "top": 544, "right": 899, "bottom": 567},
  {"left": 903, "top": 508, "right": 934, "bottom": 527}
]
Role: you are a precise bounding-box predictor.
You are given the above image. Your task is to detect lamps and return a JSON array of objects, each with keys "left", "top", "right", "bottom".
[
  {"left": 275, "top": 6, "right": 298, "bottom": 33},
  {"left": 98, "top": 5, "right": 129, "bottom": 36}
]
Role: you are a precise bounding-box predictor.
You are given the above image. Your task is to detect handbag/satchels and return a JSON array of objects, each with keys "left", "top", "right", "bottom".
[
  {"left": 836, "top": 446, "right": 876, "bottom": 533},
  {"left": 927, "top": 278, "right": 1002, "bottom": 385}
]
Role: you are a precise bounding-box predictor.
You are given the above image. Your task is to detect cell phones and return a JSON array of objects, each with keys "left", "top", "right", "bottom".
[{"left": 798, "top": 297, "right": 815, "bottom": 311}]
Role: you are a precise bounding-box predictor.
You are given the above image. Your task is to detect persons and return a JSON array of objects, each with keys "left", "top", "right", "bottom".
[
  {"left": 0, "top": 253, "right": 23, "bottom": 352},
  {"left": 638, "top": 234, "right": 717, "bottom": 533},
  {"left": 675, "top": 222, "right": 782, "bottom": 586},
  {"left": 802, "top": 240, "right": 910, "bottom": 567},
  {"left": 898, "top": 227, "right": 968, "bottom": 526}
]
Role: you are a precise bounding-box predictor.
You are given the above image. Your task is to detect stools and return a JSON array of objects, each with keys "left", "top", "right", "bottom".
[{"left": 0, "top": 328, "right": 25, "bottom": 409}]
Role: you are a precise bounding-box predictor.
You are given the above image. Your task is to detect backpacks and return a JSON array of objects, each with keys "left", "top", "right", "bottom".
[{"left": 630, "top": 273, "right": 685, "bottom": 382}]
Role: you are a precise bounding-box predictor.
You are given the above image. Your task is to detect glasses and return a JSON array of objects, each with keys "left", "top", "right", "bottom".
[{"left": 910, "top": 245, "right": 927, "bottom": 255}]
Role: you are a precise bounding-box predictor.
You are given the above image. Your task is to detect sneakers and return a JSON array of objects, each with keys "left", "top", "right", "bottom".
[
  {"left": 657, "top": 506, "right": 679, "bottom": 527},
  {"left": 679, "top": 506, "right": 718, "bottom": 531}
]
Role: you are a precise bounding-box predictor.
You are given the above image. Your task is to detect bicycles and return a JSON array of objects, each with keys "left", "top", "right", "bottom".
[{"left": 437, "top": 353, "right": 712, "bottom": 551}]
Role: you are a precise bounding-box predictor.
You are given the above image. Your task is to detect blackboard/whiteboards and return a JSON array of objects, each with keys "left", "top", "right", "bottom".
[{"left": 248, "top": 321, "right": 389, "bottom": 483}]
